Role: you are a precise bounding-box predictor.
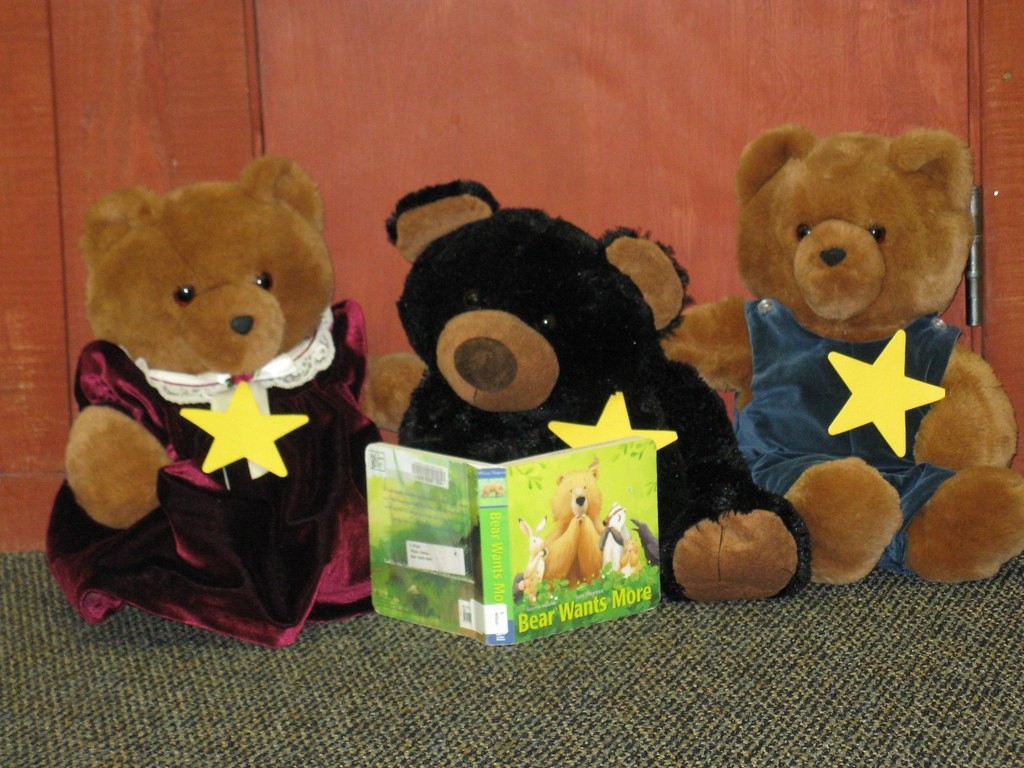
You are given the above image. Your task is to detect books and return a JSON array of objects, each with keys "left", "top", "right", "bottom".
[{"left": 365, "top": 438, "right": 658, "bottom": 645}]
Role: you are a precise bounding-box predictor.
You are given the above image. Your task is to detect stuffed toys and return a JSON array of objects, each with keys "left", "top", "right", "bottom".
[
  {"left": 384, "top": 179, "right": 811, "bottom": 601},
  {"left": 46, "top": 155, "right": 428, "bottom": 647},
  {"left": 660, "top": 125, "right": 1023, "bottom": 584}
]
